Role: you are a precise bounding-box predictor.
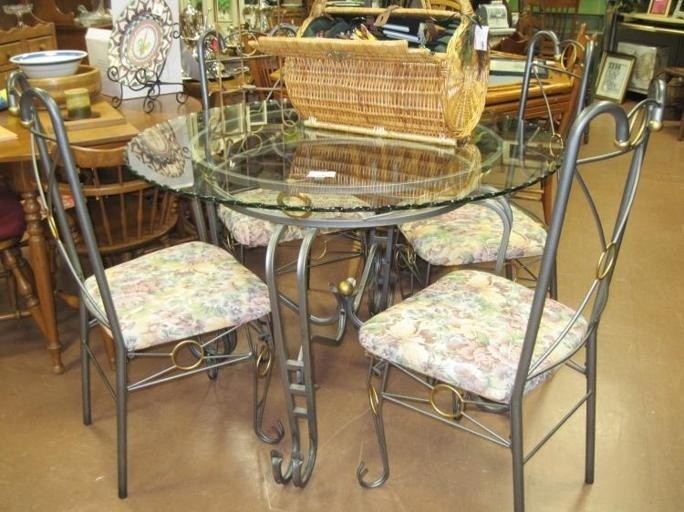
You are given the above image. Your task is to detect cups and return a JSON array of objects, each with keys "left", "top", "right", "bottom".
[{"left": 64, "top": 88, "right": 91, "bottom": 120}]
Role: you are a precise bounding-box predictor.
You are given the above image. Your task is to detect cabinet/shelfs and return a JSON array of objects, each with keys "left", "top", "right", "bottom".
[{"left": 0, "top": 22, "right": 58, "bottom": 89}]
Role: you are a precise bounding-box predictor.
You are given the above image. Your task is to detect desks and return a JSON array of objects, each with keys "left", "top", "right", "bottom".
[
  {"left": 598, "top": 10, "right": 683, "bottom": 96},
  {"left": 0, "top": 93, "right": 203, "bottom": 373}
]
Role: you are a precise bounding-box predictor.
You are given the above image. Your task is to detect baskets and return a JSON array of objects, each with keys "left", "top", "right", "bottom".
[
  {"left": 285, "top": 138, "right": 482, "bottom": 204},
  {"left": 245, "top": 0, "right": 492, "bottom": 147}
]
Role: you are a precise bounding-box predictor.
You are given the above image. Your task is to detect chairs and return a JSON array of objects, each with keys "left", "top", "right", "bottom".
[
  {"left": 356, "top": 77, "right": 663, "bottom": 510},
  {"left": 0, "top": 23, "right": 604, "bottom": 501},
  {"left": 516, "top": 2, "right": 579, "bottom": 58}
]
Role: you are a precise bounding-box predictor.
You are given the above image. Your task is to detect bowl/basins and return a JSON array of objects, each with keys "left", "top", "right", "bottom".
[{"left": 9, "top": 50, "right": 88, "bottom": 78}]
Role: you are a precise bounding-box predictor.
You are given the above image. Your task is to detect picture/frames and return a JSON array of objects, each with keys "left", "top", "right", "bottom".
[
  {"left": 594, "top": 50, "right": 636, "bottom": 105},
  {"left": 645, "top": 0, "right": 671, "bottom": 16}
]
